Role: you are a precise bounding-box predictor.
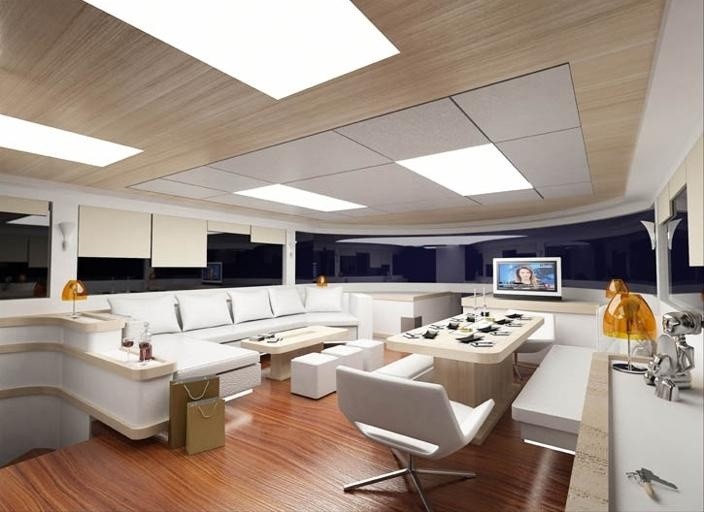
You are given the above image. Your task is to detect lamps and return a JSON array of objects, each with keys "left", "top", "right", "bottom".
[
  {"left": 602, "top": 294, "right": 658, "bottom": 373},
  {"left": 641, "top": 222, "right": 656, "bottom": 249},
  {"left": 59, "top": 221, "right": 76, "bottom": 251},
  {"left": 317, "top": 275, "right": 327, "bottom": 288},
  {"left": 668, "top": 217, "right": 683, "bottom": 249},
  {"left": 61, "top": 279, "right": 89, "bottom": 318},
  {"left": 289, "top": 241, "right": 297, "bottom": 258},
  {"left": 605, "top": 279, "right": 629, "bottom": 298}
]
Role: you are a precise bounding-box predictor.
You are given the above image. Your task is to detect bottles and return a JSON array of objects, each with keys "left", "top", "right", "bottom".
[{"left": 138, "top": 322, "right": 154, "bottom": 363}]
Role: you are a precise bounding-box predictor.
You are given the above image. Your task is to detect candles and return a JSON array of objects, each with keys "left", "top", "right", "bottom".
[
  {"left": 473, "top": 288, "right": 477, "bottom": 307},
  {"left": 482, "top": 287, "right": 485, "bottom": 304}
]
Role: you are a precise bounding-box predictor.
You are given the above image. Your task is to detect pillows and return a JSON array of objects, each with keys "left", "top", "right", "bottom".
[
  {"left": 305, "top": 285, "right": 343, "bottom": 313},
  {"left": 266, "top": 286, "right": 308, "bottom": 316},
  {"left": 175, "top": 288, "right": 233, "bottom": 332},
  {"left": 105, "top": 292, "right": 182, "bottom": 335},
  {"left": 228, "top": 287, "right": 274, "bottom": 324}
]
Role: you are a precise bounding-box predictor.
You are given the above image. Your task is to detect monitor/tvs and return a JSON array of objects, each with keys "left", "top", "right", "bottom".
[{"left": 493, "top": 257, "right": 562, "bottom": 299}]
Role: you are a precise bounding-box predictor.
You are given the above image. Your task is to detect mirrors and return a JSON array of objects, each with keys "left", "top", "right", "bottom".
[{"left": 663, "top": 182, "right": 704, "bottom": 329}]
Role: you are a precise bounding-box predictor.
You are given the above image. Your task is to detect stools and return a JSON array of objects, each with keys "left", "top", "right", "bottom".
[
  {"left": 289, "top": 352, "right": 340, "bottom": 401},
  {"left": 322, "top": 345, "right": 363, "bottom": 370},
  {"left": 346, "top": 338, "right": 384, "bottom": 373}
]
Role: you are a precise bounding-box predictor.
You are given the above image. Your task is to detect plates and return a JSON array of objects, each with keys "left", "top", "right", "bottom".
[{"left": 403, "top": 312, "right": 534, "bottom": 346}]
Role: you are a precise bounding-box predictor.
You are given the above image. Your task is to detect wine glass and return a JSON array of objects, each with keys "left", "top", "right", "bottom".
[{"left": 121, "top": 336, "right": 134, "bottom": 355}]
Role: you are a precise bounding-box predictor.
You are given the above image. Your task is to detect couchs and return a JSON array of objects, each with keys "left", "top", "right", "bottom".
[{"left": 84, "top": 284, "right": 374, "bottom": 408}]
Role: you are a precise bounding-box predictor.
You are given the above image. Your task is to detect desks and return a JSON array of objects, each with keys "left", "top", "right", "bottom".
[{"left": 563, "top": 352, "right": 703, "bottom": 511}]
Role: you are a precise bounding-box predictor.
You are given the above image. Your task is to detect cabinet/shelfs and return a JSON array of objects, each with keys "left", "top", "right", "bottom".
[{"left": 459, "top": 290, "right": 600, "bottom": 368}]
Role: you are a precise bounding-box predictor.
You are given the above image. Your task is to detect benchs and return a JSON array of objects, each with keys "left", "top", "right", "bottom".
[
  {"left": 372, "top": 352, "right": 433, "bottom": 382},
  {"left": 512, "top": 343, "right": 596, "bottom": 455}
]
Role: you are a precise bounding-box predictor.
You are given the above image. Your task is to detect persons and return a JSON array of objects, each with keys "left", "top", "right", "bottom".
[{"left": 514, "top": 266, "right": 539, "bottom": 290}]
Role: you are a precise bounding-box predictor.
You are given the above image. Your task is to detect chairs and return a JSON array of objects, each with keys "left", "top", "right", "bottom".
[
  {"left": 506, "top": 310, "right": 556, "bottom": 381},
  {"left": 335, "top": 363, "right": 496, "bottom": 512}
]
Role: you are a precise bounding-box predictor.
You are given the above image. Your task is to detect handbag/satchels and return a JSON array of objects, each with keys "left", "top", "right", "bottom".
[
  {"left": 169, "top": 373, "right": 220, "bottom": 450},
  {"left": 185, "top": 396, "right": 226, "bottom": 456}
]
